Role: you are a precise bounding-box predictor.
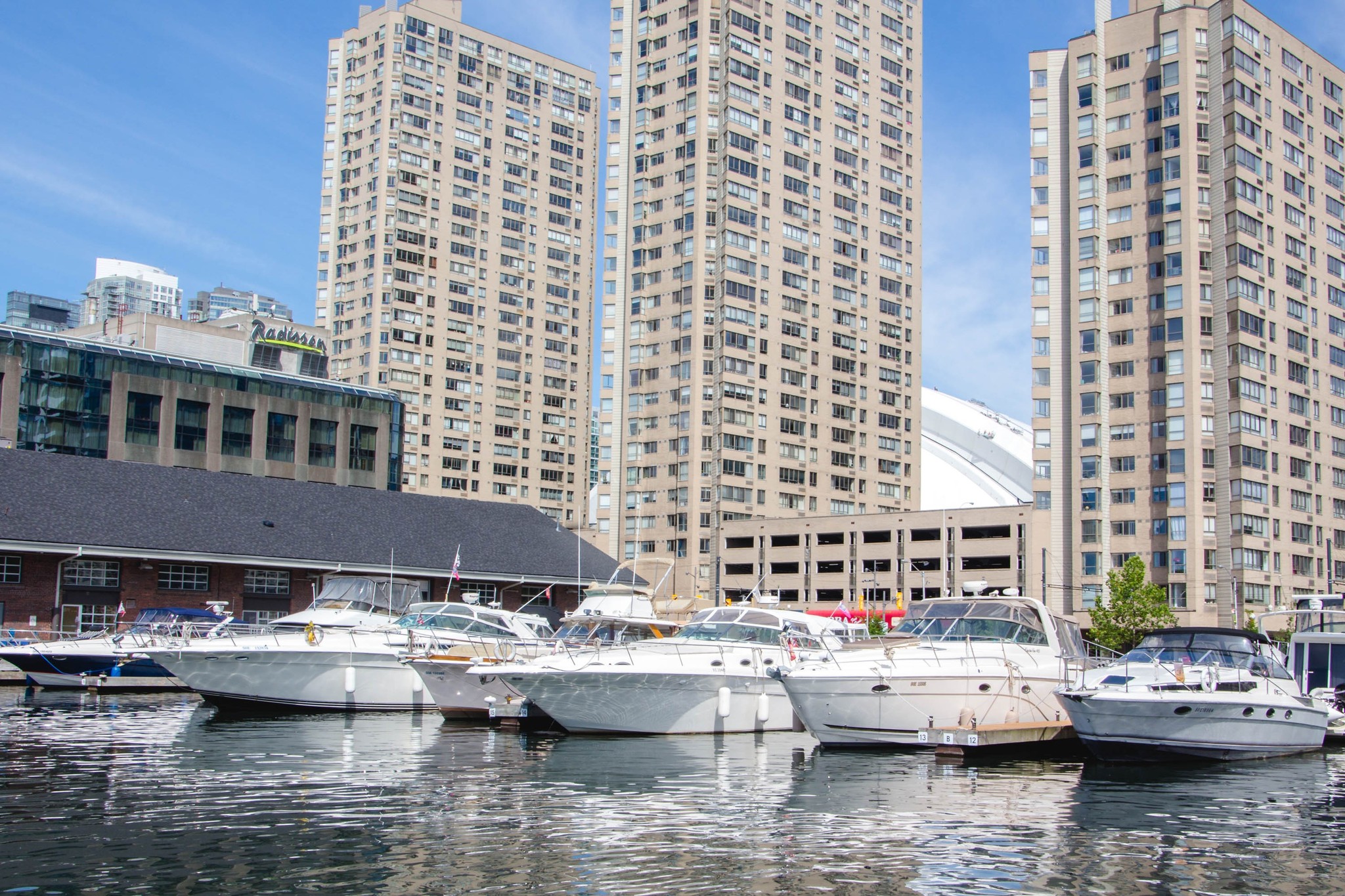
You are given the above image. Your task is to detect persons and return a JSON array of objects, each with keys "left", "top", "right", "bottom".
[
  {"left": 745, "top": 631, "right": 756, "bottom": 642},
  {"left": 1003, "top": 627, "right": 1017, "bottom": 642},
  {"left": 906, "top": 608, "right": 938, "bottom": 640},
  {"left": 588, "top": 632, "right": 598, "bottom": 646}
]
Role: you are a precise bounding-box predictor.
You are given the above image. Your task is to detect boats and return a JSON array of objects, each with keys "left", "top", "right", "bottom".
[
  {"left": 1056, "top": 626, "right": 1328, "bottom": 761},
  {"left": 2, "top": 604, "right": 244, "bottom": 684},
  {"left": 140, "top": 575, "right": 435, "bottom": 710},
  {"left": 401, "top": 616, "right": 539, "bottom": 719},
  {"left": 780, "top": 579, "right": 1085, "bottom": 744},
  {"left": 471, "top": 604, "right": 847, "bottom": 736}
]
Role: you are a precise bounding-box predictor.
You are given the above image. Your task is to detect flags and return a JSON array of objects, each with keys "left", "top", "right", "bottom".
[
  {"left": 787, "top": 638, "right": 796, "bottom": 661},
  {"left": 417, "top": 611, "right": 424, "bottom": 624},
  {"left": 452, "top": 553, "right": 461, "bottom": 580},
  {"left": 545, "top": 586, "right": 550, "bottom": 599},
  {"left": 750, "top": 586, "right": 762, "bottom": 602},
  {"left": 118, "top": 601, "right": 126, "bottom": 617},
  {"left": 838, "top": 599, "right": 851, "bottom": 618}
]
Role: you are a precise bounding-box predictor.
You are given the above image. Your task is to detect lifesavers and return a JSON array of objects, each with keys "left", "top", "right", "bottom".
[
  {"left": 305, "top": 625, "right": 324, "bottom": 646},
  {"left": 555, "top": 640, "right": 566, "bottom": 654},
  {"left": 221, "top": 631, "right": 237, "bottom": 636},
  {"left": 493, "top": 640, "right": 516, "bottom": 661},
  {"left": 1201, "top": 668, "right": 1216, "bottom": 693},
  {"left": 424, "top": 639, "right": 439, "bottom": 656}
]
